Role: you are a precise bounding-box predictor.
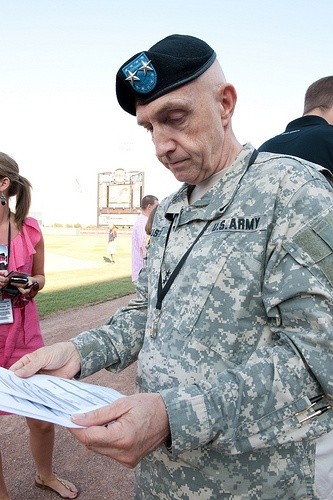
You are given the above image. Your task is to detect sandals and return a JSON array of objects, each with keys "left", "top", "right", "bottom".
[{"left": 35, "top": 471, "right": 79, "bottom": 500}]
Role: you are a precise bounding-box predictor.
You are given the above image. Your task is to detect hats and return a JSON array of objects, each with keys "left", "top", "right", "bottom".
[{"left": 116, "top": 34, "right": 216, "bottom": 117}]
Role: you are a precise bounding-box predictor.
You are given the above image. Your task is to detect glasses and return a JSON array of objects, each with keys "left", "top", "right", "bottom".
[{"left": 13, "top": 281, "right": 39, "bottom": 308}]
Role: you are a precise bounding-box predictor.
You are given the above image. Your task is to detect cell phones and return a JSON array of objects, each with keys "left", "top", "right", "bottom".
[{"left": 6, "top": 276, "right": 28, "bottom": 290}]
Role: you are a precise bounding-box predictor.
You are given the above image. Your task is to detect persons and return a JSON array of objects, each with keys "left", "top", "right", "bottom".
[
  {"left": 108, "top": 224, "right": 118, "bottom": 263},
  {"left": 131, "top": 195, "right": 158, "bottom": 283},
  {"left": 258, "top": 76, "right": 333, "bottom": 192},
  {"left": 9, "top": 34, "right": 332, "bottom": 500},
  {"left": 0, "top": 153, "right": 79, "bottom": 500}
]
